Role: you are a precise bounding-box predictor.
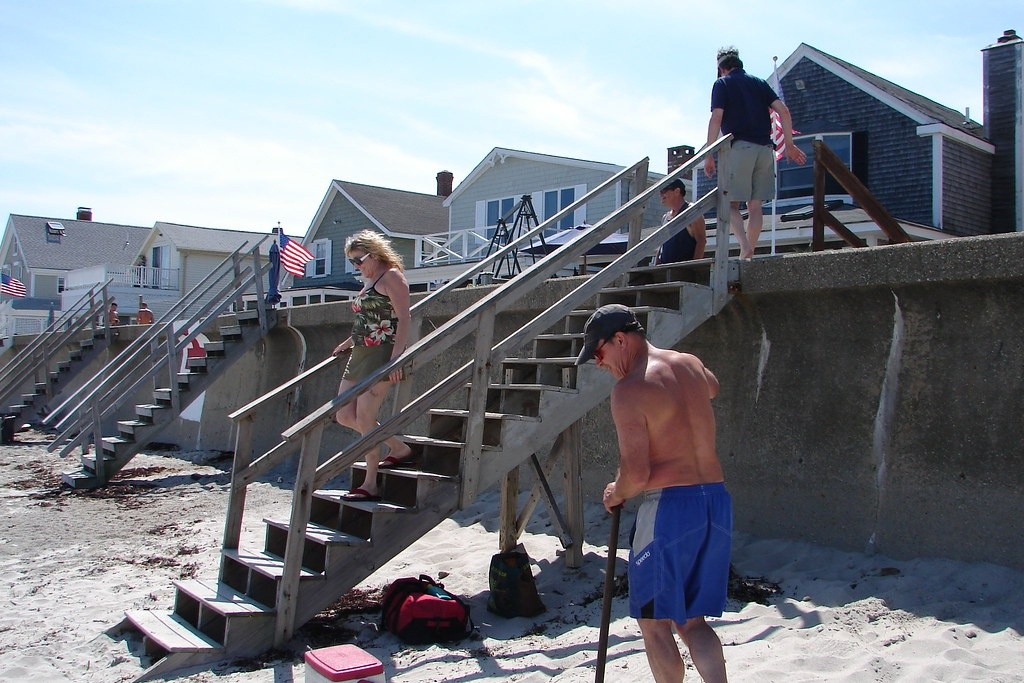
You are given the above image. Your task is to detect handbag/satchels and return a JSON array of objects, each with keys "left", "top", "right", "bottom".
[
  {"left": 485, "top": 550, "right": 547, "bottom": 617},
  {"left": 384, "top": 574, "right": 474, "bottom": 646}
]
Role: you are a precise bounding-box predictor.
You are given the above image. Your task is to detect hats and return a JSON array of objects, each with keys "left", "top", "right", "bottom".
[
  {"left": 664, "top": 179, "right": 686, "bottom": 188},
  {"left": 574, "top": 304, "right": 642, "bottom": 365},
  {"left": 717, "top": 54, "right": 740, "bottom": 79}
]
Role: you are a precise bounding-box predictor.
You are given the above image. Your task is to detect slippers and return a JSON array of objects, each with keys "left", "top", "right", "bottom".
[
  {"left": 378, "top": 450, "right": 414, "bottom": 469},
  {"left": 340, "top": 488, "right": 382, "bottom": 501}
]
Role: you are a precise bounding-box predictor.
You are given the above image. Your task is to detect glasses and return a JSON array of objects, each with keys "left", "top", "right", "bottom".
[
  {"left": 659, "top": 187, "right": 674, "bottom": 193},
  {"left": 349, "top": 251, "right": 372, "bottom": 265},
  {"left": 592, "top": 336, "right": 614, "bottom": 360}
]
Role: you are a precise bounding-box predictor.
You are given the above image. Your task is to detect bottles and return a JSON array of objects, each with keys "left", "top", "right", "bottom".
[{"left": 427, "top": 584, "right": 448, "bottom": 596}]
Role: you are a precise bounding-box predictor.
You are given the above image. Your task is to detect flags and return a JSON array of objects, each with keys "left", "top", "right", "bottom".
[
  {"left": 0, "top": 273, "right": 26, "bottom": 299},
  {"left": 769, "top": 64, "right": 801, "bottom": 160},
  {"left": 280, "top": 230, "right": 316, "bottom": 278}
]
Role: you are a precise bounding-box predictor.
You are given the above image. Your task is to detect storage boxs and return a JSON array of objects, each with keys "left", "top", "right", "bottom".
[{"left": 304, "top": 644, "right": 387, "bottom": 683}]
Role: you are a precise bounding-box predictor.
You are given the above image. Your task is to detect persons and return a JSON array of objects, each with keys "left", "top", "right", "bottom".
[
  {"left": 585, "top": 305, "right": 734, "bottom": 683},
  {"left": 333, "top": 230, "right": 412, "bottom": 500},
  {"left": 108, "top": 303, "right": 119, "bottom": 326},
  {"left": 702, "top": 47, "right": 807, "bottom": 259},
  {"left": 136, "top": 302, "right": 154, "bottom": 324},
  {"left": 656, "top": 178, "right": 707, "bottom": 264}
]
